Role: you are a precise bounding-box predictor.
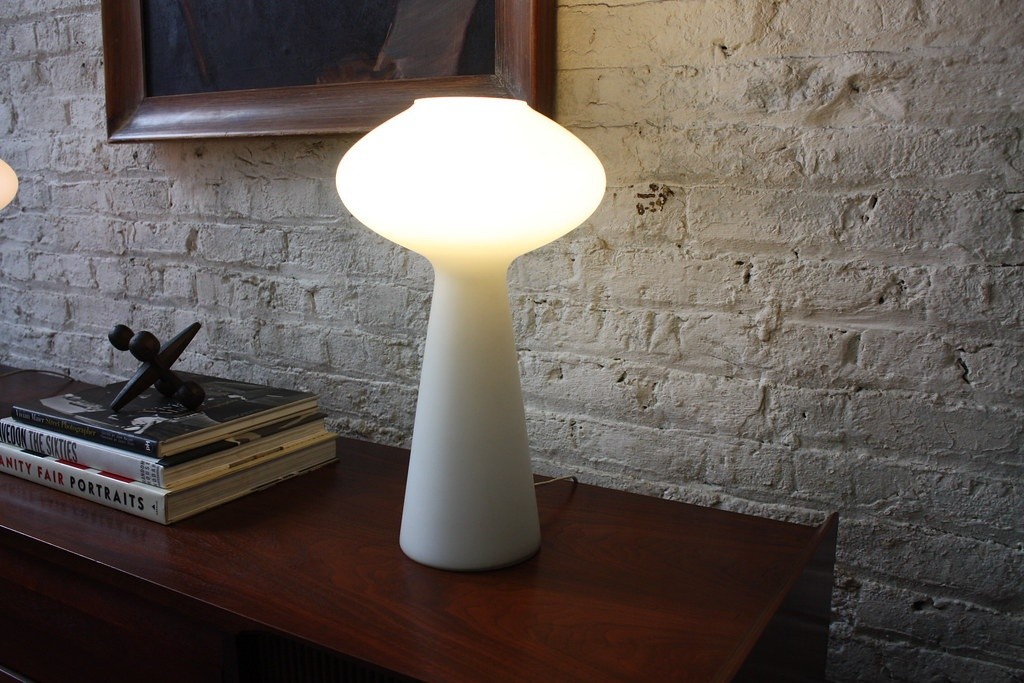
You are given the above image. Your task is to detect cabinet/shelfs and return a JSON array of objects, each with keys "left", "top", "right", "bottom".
[{"left": 0, "top": 362, "right": 840, "bottom": 683}]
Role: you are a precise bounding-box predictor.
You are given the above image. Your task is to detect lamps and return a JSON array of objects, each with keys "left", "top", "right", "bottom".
[{"left": 337, "top": 97, "right": 606, "bottom": 569}]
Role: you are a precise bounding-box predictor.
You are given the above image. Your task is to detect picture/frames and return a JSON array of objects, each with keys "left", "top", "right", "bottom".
[{"left": 98, "top": 1, "right": 558, "bottom": 145}]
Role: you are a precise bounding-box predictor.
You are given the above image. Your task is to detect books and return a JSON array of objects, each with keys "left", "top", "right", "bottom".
[{"left": 1, "top": 370, "right": 340, "bottom": 526}]
